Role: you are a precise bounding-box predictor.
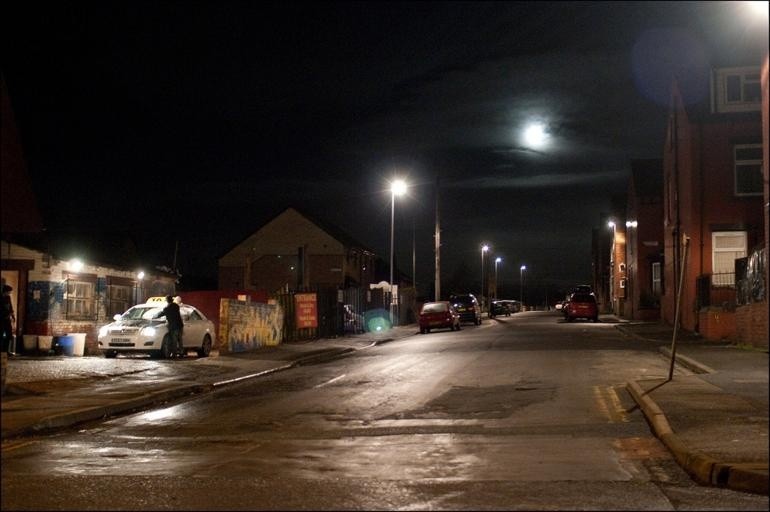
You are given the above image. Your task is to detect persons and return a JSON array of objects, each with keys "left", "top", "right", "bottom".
[
  {"left": 0, "top": 285, "right": 16, "bottom": 356},
  {"left": 152, "top": 295, "right": 184, "bottom": 359}
]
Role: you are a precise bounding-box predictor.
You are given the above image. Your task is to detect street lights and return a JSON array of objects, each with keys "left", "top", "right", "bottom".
[
  {"left": 390, "top": 178, "right": 407, "bottom": 328},
  {"left": 481, "top": 246, "right": 526, "bottom": 313}
]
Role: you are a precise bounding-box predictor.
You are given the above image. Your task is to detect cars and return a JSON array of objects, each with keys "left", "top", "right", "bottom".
[
  {"left": 419, "top": 292, "right": 481, "bottom": 334},
  {"left": 555, "top": 284, "right": 599, "bottom": 323},
  {"left": 96, "top": 295, "right": 216, "bottom": 359},
  {"left": 490, "top": 299, "right": 520, "bottom": 319}
]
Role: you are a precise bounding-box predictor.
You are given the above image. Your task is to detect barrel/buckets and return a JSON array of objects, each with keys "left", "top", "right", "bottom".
[
  {"left": 23, "top": 334, "right": 37, "bottom": 349},
  {"left": 68, "top": 333, "right": 87, "bottom": 356},
  {"left": 38, "top": 335, "right": 54, "bottom": 350},
  {"left": 54, "top": 336, "right": 73, "bottom": 356}
]
